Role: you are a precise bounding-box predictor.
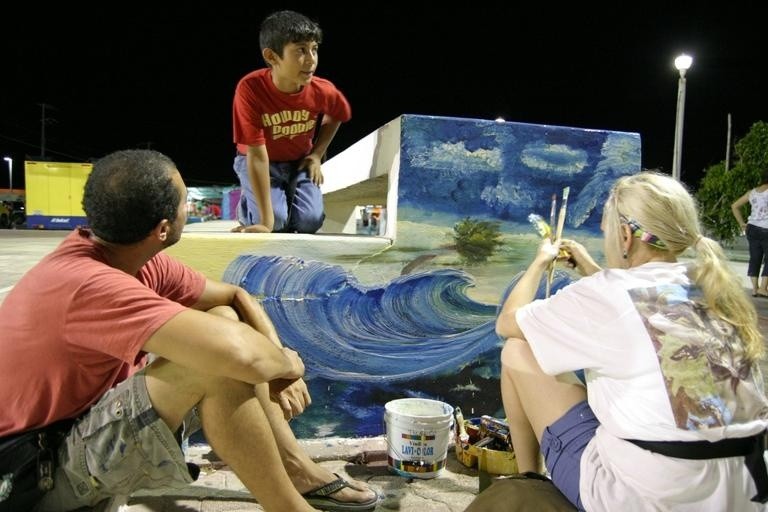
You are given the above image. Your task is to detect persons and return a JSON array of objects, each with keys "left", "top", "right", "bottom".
[
  {"left": 368, "top": 204, "right": 380, "bottom": 235},
  {"left": 379, "top": 204, "right": 387, "bottom": 237},
  {"left": 228, "top": 9, "right": 352, "bottom": 234},
  {"left": 362, "top": 203, "right": 370, "bottom": 234},
  {"left": 490, "top": 167, "right": 768, "bottom": 511},
  {"left": 187, "top": 199, "right": 221, "bottom": 222},
  {"left": 0, "top": 147, "right": 380, "bottom": 511},
  {"left": 731, "top": 181, "right": 767, "bottom": 300}
]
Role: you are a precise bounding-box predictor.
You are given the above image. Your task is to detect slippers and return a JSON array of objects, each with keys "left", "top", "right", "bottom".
[{"left": 302, "top": 473, "right": 381, "bottom": 512}]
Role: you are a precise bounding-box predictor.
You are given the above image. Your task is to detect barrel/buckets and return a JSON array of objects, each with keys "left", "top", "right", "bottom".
[{"left": 384, "top": 397, "right": 454, "bottom": 480}]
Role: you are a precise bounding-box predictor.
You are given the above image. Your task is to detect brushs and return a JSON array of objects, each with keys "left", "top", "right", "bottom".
[{"left": 453, "top": 406, "right": 472, "bottom": 445}]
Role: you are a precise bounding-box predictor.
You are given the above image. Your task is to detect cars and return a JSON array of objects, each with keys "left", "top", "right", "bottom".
[{"left": 0, "top": 201, "right": 25, "bottom": 229}]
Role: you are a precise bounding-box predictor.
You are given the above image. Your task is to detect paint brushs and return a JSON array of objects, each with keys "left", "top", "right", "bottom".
[{"left": 527, "top": 186, "right": 577, "bottom": 298}]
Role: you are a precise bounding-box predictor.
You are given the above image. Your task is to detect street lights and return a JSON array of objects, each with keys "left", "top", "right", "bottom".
[
  {"left": 670, "top": 52, "right": 696, "bottom": 179},
  {"left": 2, "top": 155, "right": 14, "bottom": 191}
]
